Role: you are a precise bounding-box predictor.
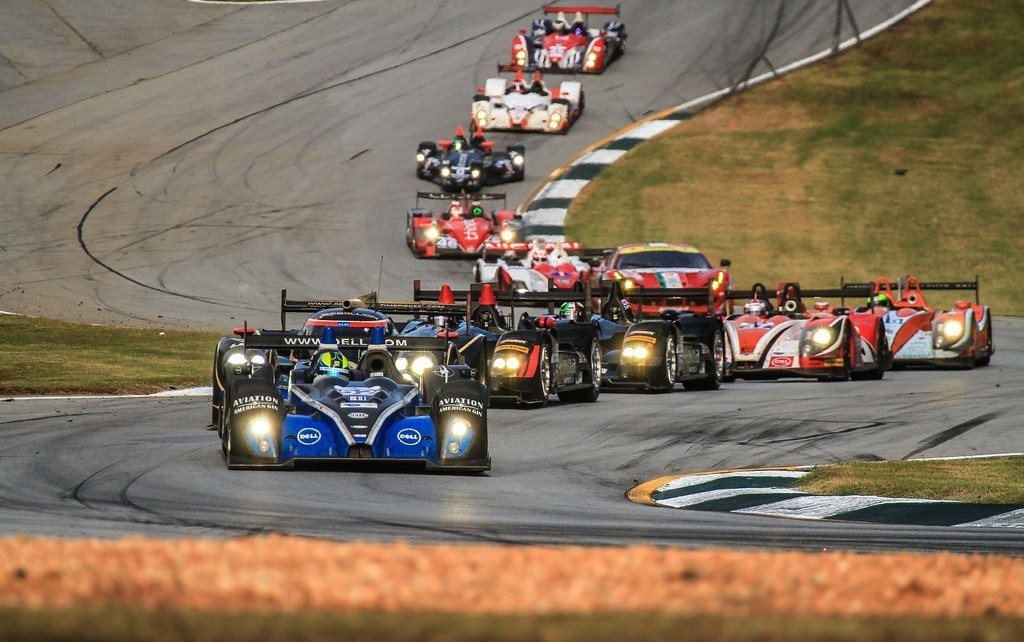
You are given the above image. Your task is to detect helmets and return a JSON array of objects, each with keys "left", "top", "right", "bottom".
[
  {"left": 866, "top": 294, "right": 890, "bottom": 309},
  {"left": 551, "top": 19, "right": 565, "bottom": 34},
  {"left": 528, "top": 248, "right": 550, "bottom": 268},
  {"left": 510, "top": 81, "right": 524, "bottom": 94},
  {"left": 449, "top": 205, "right": 464, "bottom": 216},
  {"left": 560, "top": 302, "right": 585, "bottom": 321},
  {"left": 743, "top": 299, "right": 770, "bottom": 319}
]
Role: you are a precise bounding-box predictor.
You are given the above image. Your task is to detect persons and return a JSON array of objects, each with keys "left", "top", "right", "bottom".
[
  {"left": 531, "top": 250, "right": 547, "bottom": 263},
  {"left": 867, "top": 294, "right": 889, "bottom": 307},
  {"left": 449, "top": 201, "right": 462, "bottom": 216},
  {"left": 611, "top": 299, "right": 631, "bottom": 320},
  {"left": 744, "top": 299, "right": 767, "bottom": 315},
  {"left": 512, "top": 80, "right": 524, "bottom": 93},
  {"left": 551, "top": 19, "right": 565, "bottom": 34},
  {"left": 318, "top": 351, "right": 349, "bottom": 376},
  {"left": 559, "top": 302, "right": 584, "bottom": 320},
  {"left": 452, "top": 137, "right": 463, "bottom": 150},
  {"left": 434, "top": 316, "right": 456, "bottom": 328},
  {"left": 335, "top": 328, "right": 368, "bottom": 342}
]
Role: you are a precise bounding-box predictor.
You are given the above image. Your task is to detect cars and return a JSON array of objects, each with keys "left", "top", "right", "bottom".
[{"left": 583, "top": 244, "right": 734, "bottom": 319}]
